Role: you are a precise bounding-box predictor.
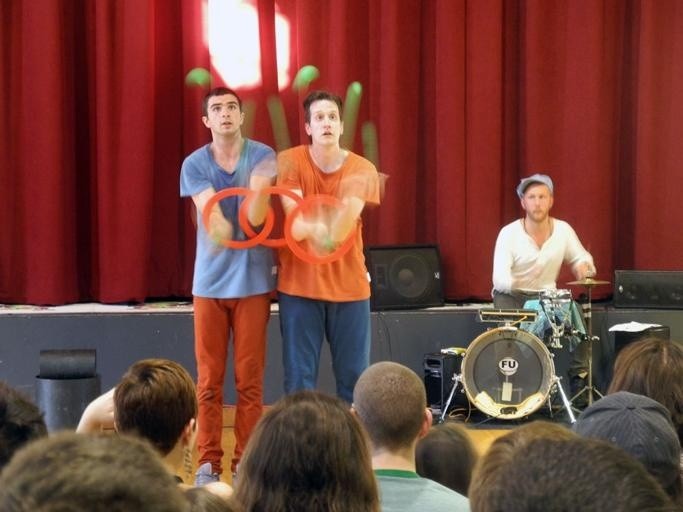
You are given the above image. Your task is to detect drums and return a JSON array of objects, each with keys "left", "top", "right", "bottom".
[{"left": 461, "top": 326, "right": 555, "bottom": 420}]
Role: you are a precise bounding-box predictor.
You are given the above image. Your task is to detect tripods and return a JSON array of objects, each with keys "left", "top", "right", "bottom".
[{"left": 554, "top": 287, "right": 607, "bottom": 417}]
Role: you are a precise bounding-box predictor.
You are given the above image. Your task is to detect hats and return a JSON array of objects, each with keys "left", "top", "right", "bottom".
[
  {"left": 572, "top": 390, "right": 680, "bottom": 488},
  {"left": 517, "top": 174, "right": 554, "bottom": 199}
]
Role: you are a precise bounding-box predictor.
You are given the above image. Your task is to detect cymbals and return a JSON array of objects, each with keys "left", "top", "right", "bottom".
[{"left": 566, "top": 278, "right": 610, "bottom": 288}]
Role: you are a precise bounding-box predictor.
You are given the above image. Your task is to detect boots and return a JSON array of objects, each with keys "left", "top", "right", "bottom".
[{"left": 571, "top": 375, "right": 591, "bottom": 409}]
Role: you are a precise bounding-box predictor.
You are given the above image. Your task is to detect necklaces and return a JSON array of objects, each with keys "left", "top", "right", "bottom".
[{"left": 522, "top": 213, "right": 554, "bottom": 243}]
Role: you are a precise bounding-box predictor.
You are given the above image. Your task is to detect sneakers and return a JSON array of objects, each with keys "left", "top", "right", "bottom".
[{"left": 194, "top": 463, "right": 219, "bottom": 487}]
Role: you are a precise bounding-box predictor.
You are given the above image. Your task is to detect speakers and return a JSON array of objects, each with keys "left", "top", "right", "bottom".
[
  {"left": 612, "top": 269, "right": 683, "bottom": 311},
  {"left": 422, "top": 352, "right": 482, "bottom": 419},
  {"left": 364, "top": 243, "right": 446, "bottom": 311}
]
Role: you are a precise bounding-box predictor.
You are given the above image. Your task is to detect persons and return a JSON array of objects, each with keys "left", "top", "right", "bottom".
[
  {"left": 491, "top": 173, "right": 597, "bottom": 409},
  {"left": 277, "top": 89, "right": 383, "bottom": 418},
  {"left": 0, "top": 334, "right": 681, "bottom": 511},
  {"left": 178, "top": 86, "right": 278, "bottom": 498}
]
